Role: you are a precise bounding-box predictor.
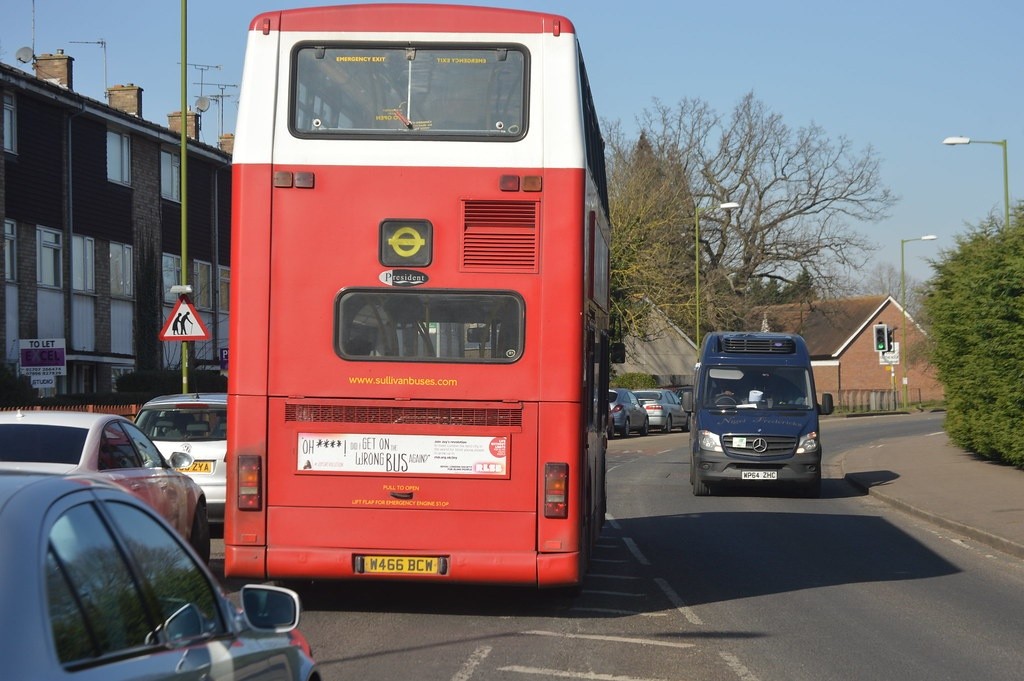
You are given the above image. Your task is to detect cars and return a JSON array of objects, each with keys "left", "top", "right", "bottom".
[
  {"left": 656, "top": 384, "right": 693, "bottom": 413},
  {"left": 0, "top": 468, "right": 326, "bottom": 680},
  {"left": 607, "top": 404, "right": 616, "bottom": 437},
  {"left": 632, "top": 388, "right": 690, "bottom": 432},
  {"left": 132, "top": 393, "right": 228, "bottom": 520},
  {"left": 0, "top": 410, "right": 213, "bottom": 565},
  {"left": 608, "top": 387, "right": 650, "bottom": 437}
]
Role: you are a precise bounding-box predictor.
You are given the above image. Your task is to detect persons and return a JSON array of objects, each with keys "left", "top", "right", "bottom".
[{"left": 711, "top": 377, "right": 744, "bottom": 405}]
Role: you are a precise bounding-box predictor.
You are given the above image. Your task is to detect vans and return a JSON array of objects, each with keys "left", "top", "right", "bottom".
[{"left": 681, "top": 330, "right": 835, "bottom": 496}]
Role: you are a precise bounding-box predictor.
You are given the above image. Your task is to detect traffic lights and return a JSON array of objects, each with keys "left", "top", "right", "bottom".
[{"left": 873, "top": 324, "right": 892, "bottom": 354}]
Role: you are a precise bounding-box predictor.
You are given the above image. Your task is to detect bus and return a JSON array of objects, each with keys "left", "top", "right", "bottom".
[{"left": 222, "top": 4, "right": 628, "bottom": 609}]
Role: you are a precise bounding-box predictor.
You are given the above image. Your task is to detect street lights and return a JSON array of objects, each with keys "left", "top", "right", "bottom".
[
  {"left": 900, "top": 235, "right": 940, "bottom": 410},
  {"left": 943, "top": 136, "right": 1010, "bottom": 232},
  {"left": 694, "top": 200, "right": 740, "bottom": 362}
]
